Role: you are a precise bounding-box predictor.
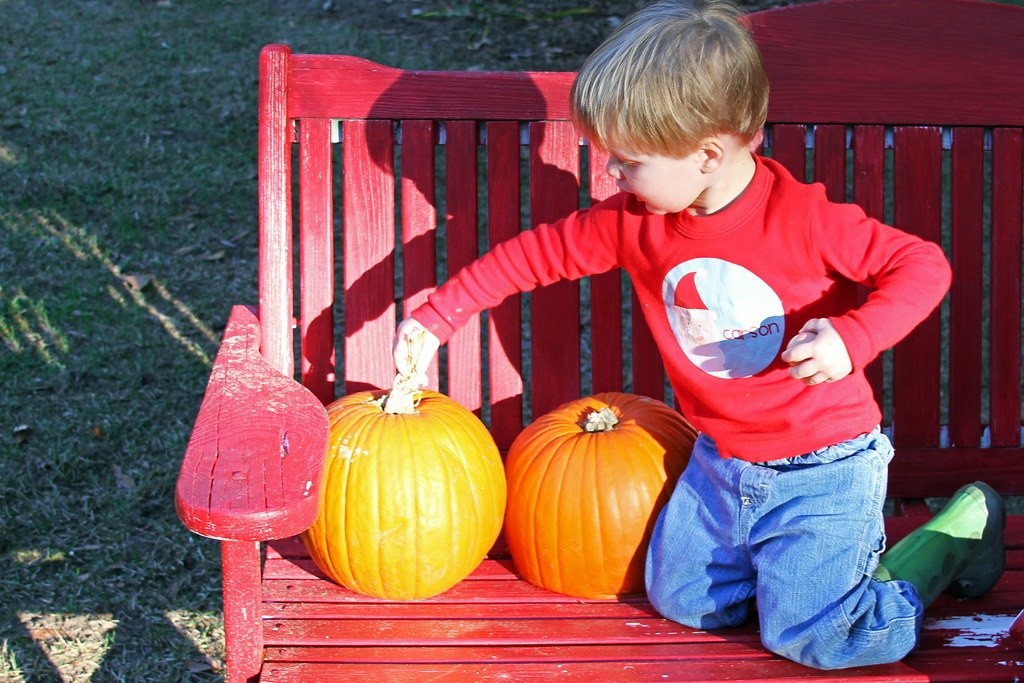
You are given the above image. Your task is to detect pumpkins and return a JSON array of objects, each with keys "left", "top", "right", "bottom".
[{"left": 301, "top": 329, "right": 705, "bottom": 601}]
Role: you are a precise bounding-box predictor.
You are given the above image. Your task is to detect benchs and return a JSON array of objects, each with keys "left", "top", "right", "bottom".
[{"left": 174, "top": 0, "right": 1024, "bottom": 683}]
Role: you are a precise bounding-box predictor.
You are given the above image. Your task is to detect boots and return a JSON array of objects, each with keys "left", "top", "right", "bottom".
[{"left": 870, "top": 481, "right": 1006, "bottom": 612}]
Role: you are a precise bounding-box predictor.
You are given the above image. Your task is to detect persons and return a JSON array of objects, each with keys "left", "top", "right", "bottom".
[{"left": 390, "top": 1, "right": 1010, "bottom": 671}]
